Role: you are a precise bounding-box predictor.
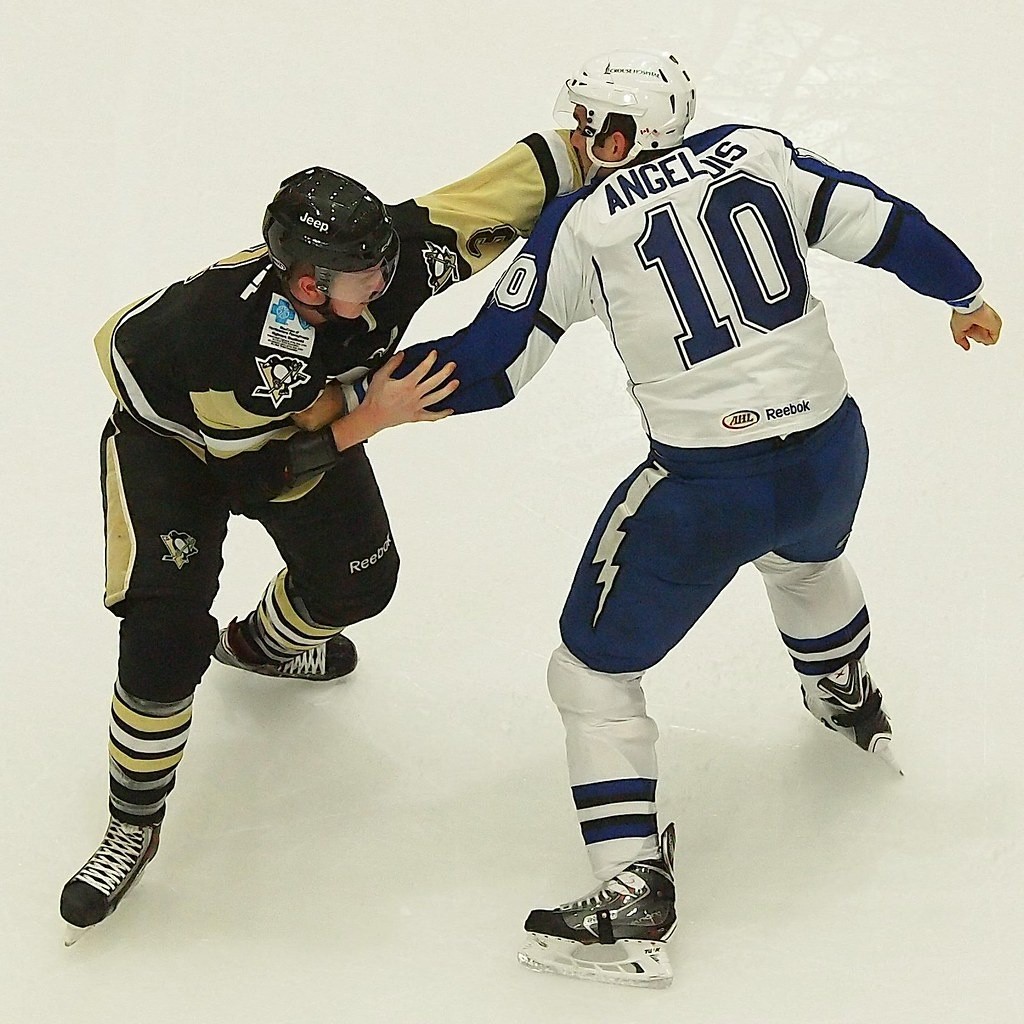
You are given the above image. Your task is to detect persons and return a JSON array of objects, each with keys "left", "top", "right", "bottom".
[
  {"left": 57, "top": 124, "right": 587, "bottom": 945},
  {"left": 290, "top": 48, "right": 1002, "bottom": 987}
]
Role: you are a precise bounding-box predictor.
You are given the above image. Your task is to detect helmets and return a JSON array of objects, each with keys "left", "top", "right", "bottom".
[
  {"left": 262, "top": 166, "right": 400, "bottom": 285},
  {"left": 552, "top": 48, "right": 696, "bottom": 168}
]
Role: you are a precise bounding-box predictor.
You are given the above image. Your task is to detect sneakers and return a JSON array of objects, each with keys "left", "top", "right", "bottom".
[
  {"left": 60, "top": 816, "right": 162, "bottom": 946},
  {"left": 801, "top": 659, "right": 906, "bottom": 776},
  {"left": 518, "top": 823, "right": 678, "bottom": 989},
  {"left": 213, "top": 611, "right": 357, "bottom": 682}
]
